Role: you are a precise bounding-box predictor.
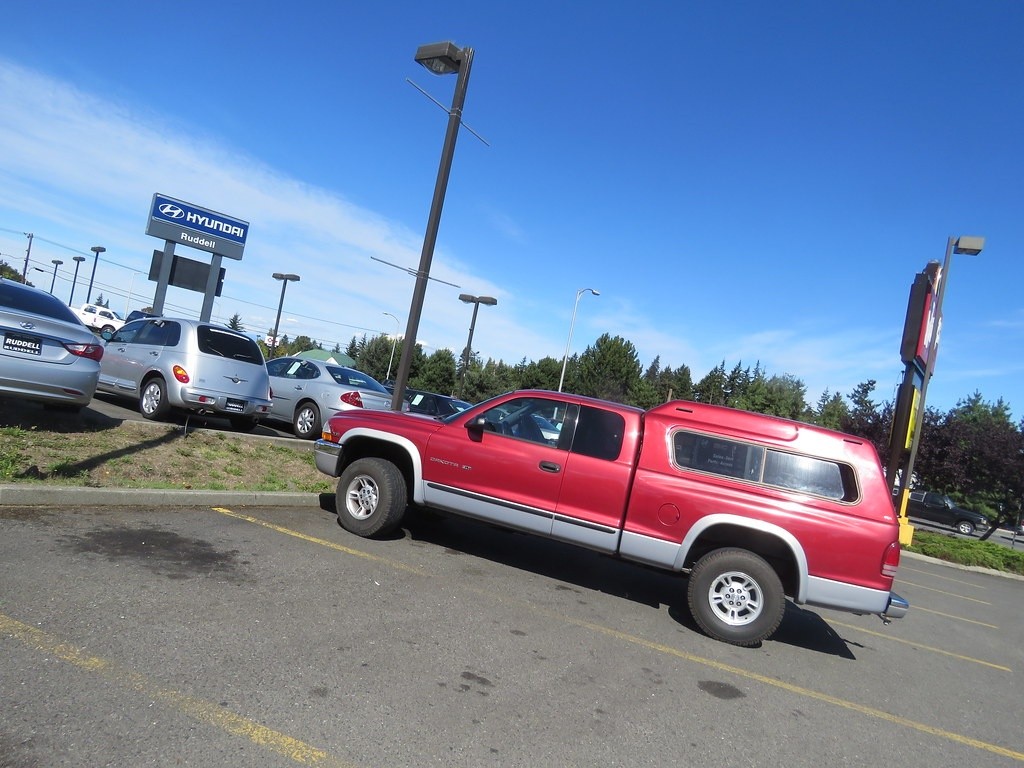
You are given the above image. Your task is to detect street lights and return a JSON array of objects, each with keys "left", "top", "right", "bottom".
[
  {"left": 125, "top": 271, "right": 140, "bottom": 320},
  {"left": 894, "top": 236, "right": 986, "bottom": 519},
  {"left": 269, "top": 272, "right": 300, "bottom": 360},
  {"left": 86, "top": 246, "right": 106, "bottom": 303},
  {"left": 68, "top": 256, "right": 85, "bottom": 306},
  {"left": 457, "top": 293, "right": 497, "bottom": 399},
  {"left": 383, "top": 312, "right": 400, "bottom": 379},
  {"left": 391, "top": 40, "right": 476, "bottom": 412},
  {"left": 553, "top": 286, "right": 600, "bottom": 417},
  {"left": 50, "top": 260, "right": 63, "bottom": 294}
]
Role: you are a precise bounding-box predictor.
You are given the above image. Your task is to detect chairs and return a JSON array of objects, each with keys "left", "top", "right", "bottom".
[{"left": 298, "top": 368, "right": 310, "bottom": 379}]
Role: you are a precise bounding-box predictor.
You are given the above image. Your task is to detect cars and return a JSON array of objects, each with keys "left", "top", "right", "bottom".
[
  {"left": 0, "top": 276, "right": 105, "bottom": 414},
  {"left": 95, "top": 317, "right": 274, "bottom": 434},
  {"left": 263, "top": 355, "right": 411, "bottom": 440},
  {"left": 382, "top": 379, "right": 567, "bottom": 447}
]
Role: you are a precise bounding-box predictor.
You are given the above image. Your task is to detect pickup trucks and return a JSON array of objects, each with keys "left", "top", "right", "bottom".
[
  {"left": 313, "top": 389, "right": 909, "bottom": 648},
  {"left": 893, "top": 485, "right": 989, "bottom": 536},
  {"left": 69, "top": 303, "right": 126, "bottom": 337}
]
